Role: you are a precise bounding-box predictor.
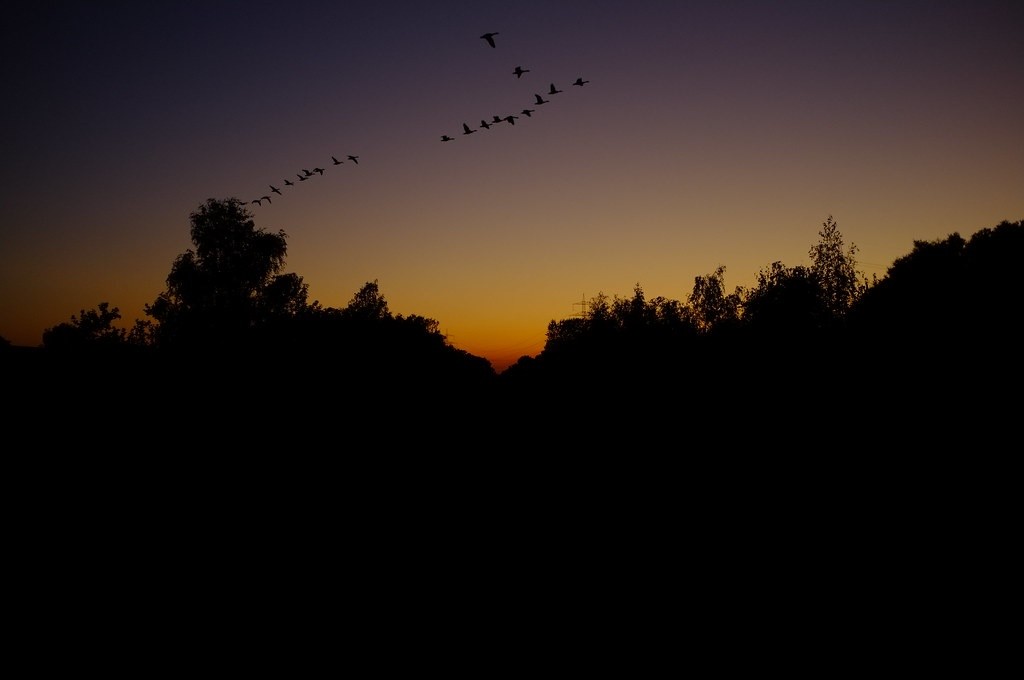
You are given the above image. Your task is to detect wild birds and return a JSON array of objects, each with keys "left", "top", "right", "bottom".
[
  {"left": 479, "top": 32, "right": 500, "bottom": 49},
  {"left": 237, "top": 153, "right": 365, "bottom": 207},
  {"left": 512, "top": 66, "right": 531, "bottom": 79},
  {"left": 438, "top": 76, "right": 591, "bottom": 142}
]
